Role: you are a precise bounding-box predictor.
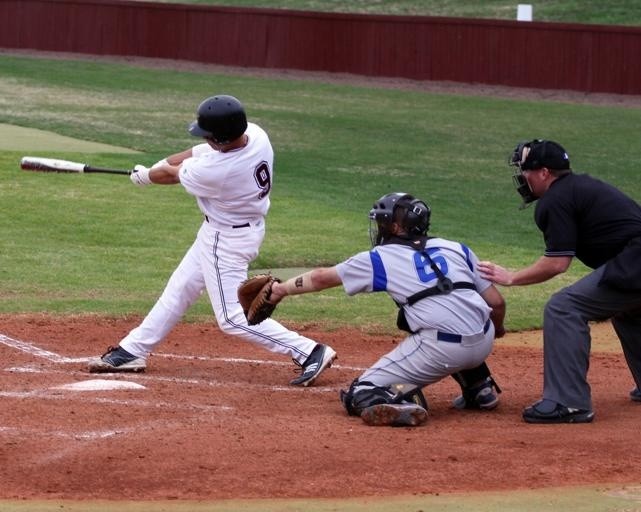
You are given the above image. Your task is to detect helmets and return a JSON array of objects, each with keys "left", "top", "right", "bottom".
[
  {"left": 368, "top": 192, "right": 431, "bottom": 248},
  {"left": 186, "top": 94, "right": 247, "bottom": 144}
]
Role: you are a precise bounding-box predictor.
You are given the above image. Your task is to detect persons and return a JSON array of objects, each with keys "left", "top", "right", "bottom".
[
  {"left": 272, "top": 191, "right": 505, "bottom": 428},
  {"left": 477, "top": 138, "right": 641, "bottom": 423},
  {"left": 88, "top": 95, "right": 337, "bottom": 388}
]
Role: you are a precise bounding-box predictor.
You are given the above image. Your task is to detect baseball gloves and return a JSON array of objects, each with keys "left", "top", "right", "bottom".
[{"left": 237, "top": 274, "right": 282, "bottom": 326}]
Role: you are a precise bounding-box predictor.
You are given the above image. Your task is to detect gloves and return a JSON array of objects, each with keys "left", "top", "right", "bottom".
[{"left": 130, "top": 164, "right": 151, "bottom": 187}]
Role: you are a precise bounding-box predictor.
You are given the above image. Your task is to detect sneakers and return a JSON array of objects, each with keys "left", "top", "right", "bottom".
[
  {"left": 522, "top": 400, "right": 594, "bottom": 423},
  {"left": 360, "top": 402, "right": 429, "bottom": 427},
  {"left": 87, "top": 345, "right": 147, "bottom": 373},
  {"left": 630, "top": 388, "right": 640, "bottom": 401},
  {"left": 289, "top": 343, "right": 338, "bottom": 387},
  {"left": 452, "top": 380, "right": 499, "bottom": 410}
]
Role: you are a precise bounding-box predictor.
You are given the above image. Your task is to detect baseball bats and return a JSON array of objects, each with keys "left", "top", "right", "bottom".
[{"left": 20, "top": 156, "right": 137, "bottom": 174}]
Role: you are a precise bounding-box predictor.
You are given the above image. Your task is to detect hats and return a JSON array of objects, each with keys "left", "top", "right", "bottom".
[{"left": 508, "top": 139, "right": 569, "bottom": 211}]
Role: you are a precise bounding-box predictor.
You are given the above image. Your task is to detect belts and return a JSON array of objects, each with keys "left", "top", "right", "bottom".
[
  {"left": 437, "top": 318, "right": 490, "bottom": 343},
  {"left": 205, "top": 215, "right": 250, "bottom": 228}
]
